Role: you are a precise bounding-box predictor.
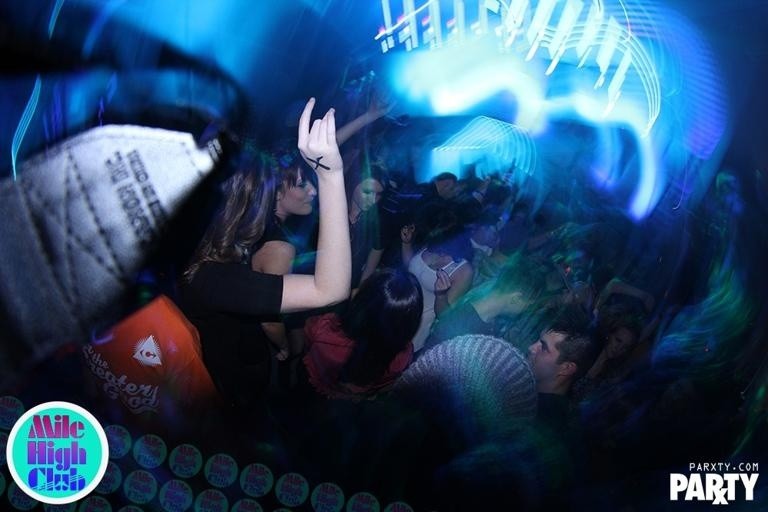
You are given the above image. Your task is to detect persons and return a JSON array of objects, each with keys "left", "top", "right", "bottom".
[{"left": 139, "top": 95, "right": 691, "bottom": 433}]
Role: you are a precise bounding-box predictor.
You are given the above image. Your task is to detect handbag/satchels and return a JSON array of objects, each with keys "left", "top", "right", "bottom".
[{"left": 82, "top": 294, "right": 220, "bottom": 435}]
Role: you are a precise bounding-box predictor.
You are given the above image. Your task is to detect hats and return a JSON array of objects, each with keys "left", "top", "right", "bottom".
[{"left": 383, "top": 335, "right": 537, "bottom": 475}]
